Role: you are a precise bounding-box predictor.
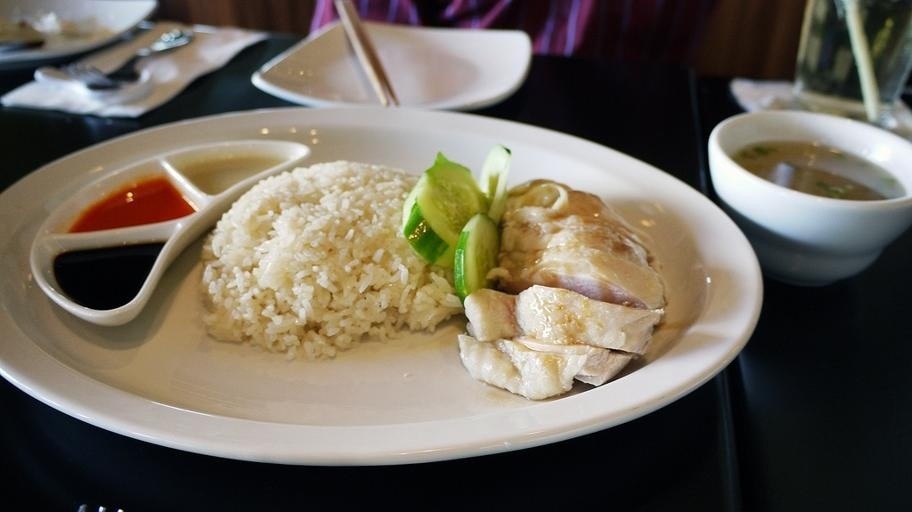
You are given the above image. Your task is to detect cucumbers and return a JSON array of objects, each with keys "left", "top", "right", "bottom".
[{"left": 401, "top": 145, "right": 513, "bottom": 307}]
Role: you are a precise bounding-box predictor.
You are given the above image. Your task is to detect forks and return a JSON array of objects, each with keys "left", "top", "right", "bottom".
[{"left": 70, "top": 29, "right": 196, "bottom": 95}]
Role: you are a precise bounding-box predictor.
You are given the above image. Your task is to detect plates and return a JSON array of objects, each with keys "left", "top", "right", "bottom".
[
  {"left": 249, "top": 17, "right": 535, "bottom": 113},
  {"left": 1, "top": 21, "right": 270, "bottom": 120},
  {"left": 1, "top": 1, "right": 158, "bottom": 69},
  {"left": 3, "top": 103, "right": 766, "bottom": 469},
  {"left": 729, "top": 75, "right": 911, "bottom": 142},
  {"left": 29, "top": 137, "right": 314, "bottom": 328}
]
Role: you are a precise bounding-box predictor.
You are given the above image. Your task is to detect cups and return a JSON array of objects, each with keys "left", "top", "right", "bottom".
[{"left": 792, "top": 0, "right": 911, "bottom": 129}]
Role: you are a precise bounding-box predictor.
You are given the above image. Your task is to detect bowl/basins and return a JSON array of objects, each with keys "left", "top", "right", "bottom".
[{"left": 707, "top": 109, "right": 912, "bottom": 291}]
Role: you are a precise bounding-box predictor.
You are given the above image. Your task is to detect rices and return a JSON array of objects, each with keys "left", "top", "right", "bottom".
[{"left": 198, "top": 159, "right": 465, "bottom": 357}]
